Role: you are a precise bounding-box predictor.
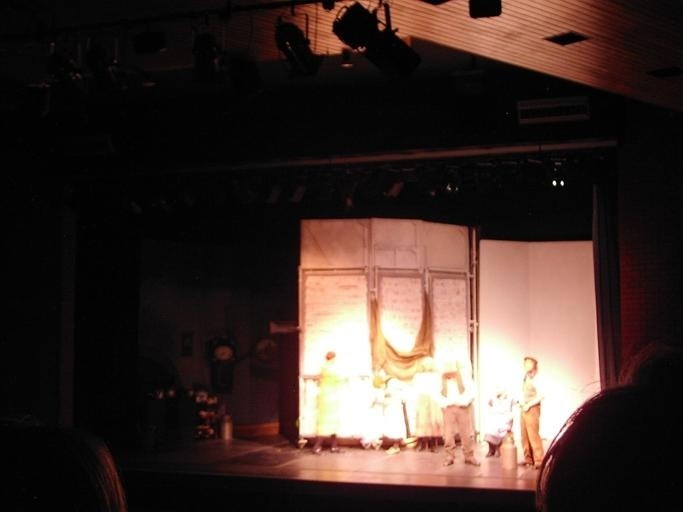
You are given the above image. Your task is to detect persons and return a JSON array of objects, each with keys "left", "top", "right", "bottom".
[
  {"left": 518, "top": 357, "right": 544, "bottom": 469},
  {"left": 484, "top": 392, "right": 520, "bottom": 456},
  {"left": 0, "top": 416, "right": 128, "bottom": 512},
  {"left": 534, "top": 338, "right": 683, "bottom": 512},
  {"left": 441, "top": 359, "right": 480, "bottom": 466}
]
